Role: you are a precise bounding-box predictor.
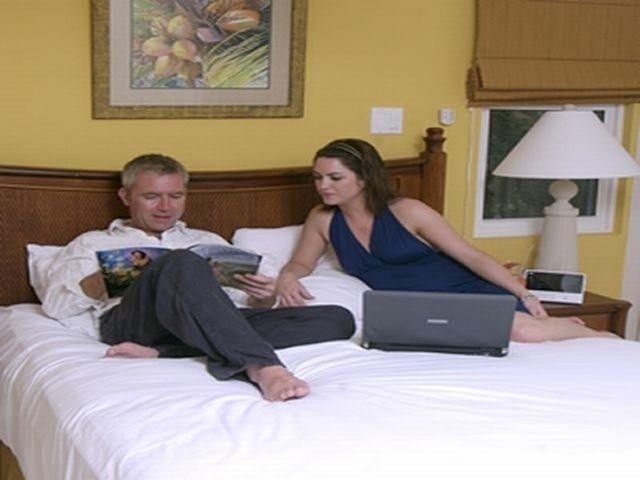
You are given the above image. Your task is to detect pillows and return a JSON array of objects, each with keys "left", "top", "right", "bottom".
[
  {"left": 24, "top": 243, "right": 104, "bottom": 344},
  {"left": 230, "top": 224, "right": 376, "bottom": 342}
]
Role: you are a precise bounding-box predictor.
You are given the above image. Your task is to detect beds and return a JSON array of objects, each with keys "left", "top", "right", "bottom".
[{"left": 1, "top": 127, "right": 640, "bottom": 480}]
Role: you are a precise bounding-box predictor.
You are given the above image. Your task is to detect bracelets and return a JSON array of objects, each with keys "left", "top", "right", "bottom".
[{"left": 520, "top": 293, "right": 536, "bottom": 303}]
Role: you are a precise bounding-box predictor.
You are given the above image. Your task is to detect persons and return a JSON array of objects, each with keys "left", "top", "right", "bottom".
[
  {"left": 271, "top": 138, "right": 621, "bottom": 342},
  {"left": 40, "top": 155, "right": 356, "bottom": 400},
  {"left": 127, "top": 249, "right": 153, "bottom": 274}
]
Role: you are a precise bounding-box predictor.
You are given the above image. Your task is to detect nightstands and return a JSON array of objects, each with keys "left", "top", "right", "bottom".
[{"left": 540, "top": 291, "right": 633, "bottom": 340}]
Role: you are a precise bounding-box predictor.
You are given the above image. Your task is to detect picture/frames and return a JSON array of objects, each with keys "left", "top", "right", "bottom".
[
  {"left": 472, "top": 106, "right": 625, "bottom": 239},
  {"left": 89, "top": 0, "right": 308, "bottom": 120}
]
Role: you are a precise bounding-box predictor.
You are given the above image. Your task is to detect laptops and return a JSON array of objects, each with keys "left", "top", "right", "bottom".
[{"left": 362, "top": 290, "right": 517, "bottom": 356}]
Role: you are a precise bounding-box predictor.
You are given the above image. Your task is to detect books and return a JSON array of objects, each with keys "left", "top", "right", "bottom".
[{"left": 94, "top": 244, "right": 262, "bottom": 299}]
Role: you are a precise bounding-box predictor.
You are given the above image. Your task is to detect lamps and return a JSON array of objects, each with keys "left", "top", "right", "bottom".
[{"left": 492, "top": 103, "right": 640, "bottom": 275}]
